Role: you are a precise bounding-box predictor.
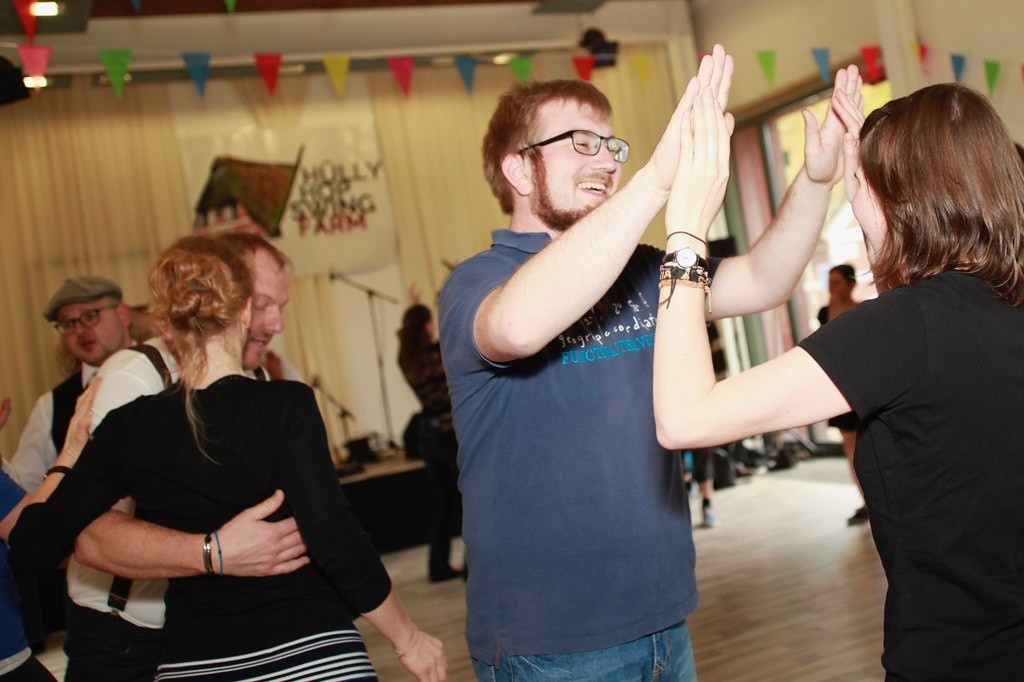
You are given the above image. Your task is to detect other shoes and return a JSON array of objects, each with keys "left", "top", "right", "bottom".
[
  {"left": 847, "top": 508, "right": 868, "bottom": 525},
  {"left": 687, "top": 480, "right": 702, "bottom": 499},
  {"left": 428, "top": 566, "right": 463, "bottom": 583}
]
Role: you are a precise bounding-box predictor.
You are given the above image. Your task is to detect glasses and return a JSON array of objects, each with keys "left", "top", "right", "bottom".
[
  {"left": 519, "top": 128, "right": 631, "bottom": 164},
  {"left": 53, "top": 304, "right": 120, "bottom": 338}
]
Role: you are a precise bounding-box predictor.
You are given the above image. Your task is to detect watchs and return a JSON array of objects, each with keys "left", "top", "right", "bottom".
[{"left": 661, "top": 248, "right": 710, "bottom": 273}]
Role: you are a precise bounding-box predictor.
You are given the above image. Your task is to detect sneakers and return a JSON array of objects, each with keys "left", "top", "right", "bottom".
[{"left": 702, "top": 509, "right": 719, "bottom": 527}]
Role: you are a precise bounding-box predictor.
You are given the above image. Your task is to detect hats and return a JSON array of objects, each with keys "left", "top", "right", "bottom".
[{"left": 43, "top": 273, "right": 123, "bottom": 321}]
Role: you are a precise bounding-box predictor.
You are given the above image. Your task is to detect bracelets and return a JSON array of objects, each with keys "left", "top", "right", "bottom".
[
  {"left": 203, "top": 533, "right": 213, "bottom": 572},
  {"left": 43, "top": 466, "right": 71, "bottom": 479},
  {"left": 658, "top": 266, "right": 712, "bottom": 312},
  {"left": 665, "top": 231, "right": 708, "bottom": 249},
  {"left": 214, "top": 530, "right": 222, "bottom": 577}
]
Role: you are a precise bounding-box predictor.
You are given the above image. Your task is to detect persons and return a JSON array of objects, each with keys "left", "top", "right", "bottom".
[
  {"left": 676, "top": 318, "right": 729, "bottom": 527},
  {"left": 8, "top": 236, "right": 448, "bottom": 682},
  {"left": 0, "top": 275, "right": 143, "bottom": 496},
  {"left": 652, "top": 83, "right": 1024, "bottom": 682},
  {"left": 62, "top": 232, "right": 302, "bottom": 682},
  {"left": 0, "top": 376, "right": 103, "bottom": 682},
  {"left": 818, "top": 265, "right": 870, "bottom": 525},
  {"left": 398, "top": 303, "right": 466, "bottom": 581},
  {"left": 436, "top": 44, "right": 866, "bottom": 682}
]
